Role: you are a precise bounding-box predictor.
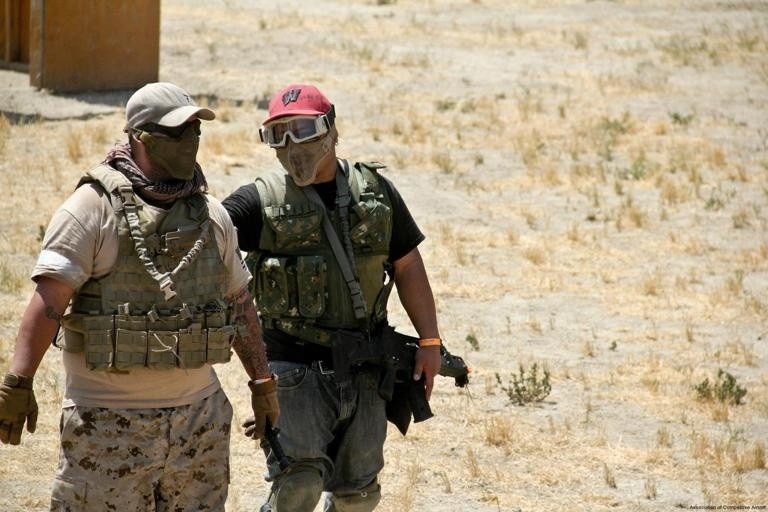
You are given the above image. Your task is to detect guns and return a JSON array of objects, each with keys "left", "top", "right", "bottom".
[{"left": 331, "top": 326, "right": 469, "bottom": 423}]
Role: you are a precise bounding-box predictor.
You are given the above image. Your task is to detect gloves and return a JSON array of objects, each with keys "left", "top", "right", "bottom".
[
  {"left": 0, "top": 374, "right": 39, "bottom": 445},
  {"left": 242, "top": 373, "right": 279, "bottom": 439}
]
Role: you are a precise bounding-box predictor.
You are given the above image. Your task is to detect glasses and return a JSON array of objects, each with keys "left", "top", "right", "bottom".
[
  {"left": 257, "top": 104, "right": 336, "bottom": 149},
  {"left": 131, "top": 120, "right": 201, "bottom": 138}
]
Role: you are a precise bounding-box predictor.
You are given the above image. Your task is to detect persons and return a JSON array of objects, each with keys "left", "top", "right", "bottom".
[
  {"left": 221, "top": 85, "right": 442, "bottom": 512},
  {"left": 1, "top": 82, "right": 281, "bottom": 511}
]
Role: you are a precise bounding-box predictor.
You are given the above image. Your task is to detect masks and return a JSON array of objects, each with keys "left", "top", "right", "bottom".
[
  {"left": 139, "top": 131, "right": 200, "bottom": 180},
  {"left": 275, "top": 126, "right": 339, "bottom": 187}
]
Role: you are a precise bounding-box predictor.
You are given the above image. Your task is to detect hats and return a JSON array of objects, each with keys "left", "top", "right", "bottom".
[
  {"left": 123, "top": 81, "right": 215, "bottom": 133},
  {"left": 262, "top": 83, "right": 331, "bottom": 123}
]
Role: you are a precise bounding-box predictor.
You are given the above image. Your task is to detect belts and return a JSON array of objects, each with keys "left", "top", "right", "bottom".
[{"left": 310, "top": 359, "right": 336, "bottom": 375}]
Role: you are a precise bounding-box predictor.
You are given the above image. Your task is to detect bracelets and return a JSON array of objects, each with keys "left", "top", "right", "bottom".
[{"left": 418, "top": 338, "right": 443, "bottom": 347}]
